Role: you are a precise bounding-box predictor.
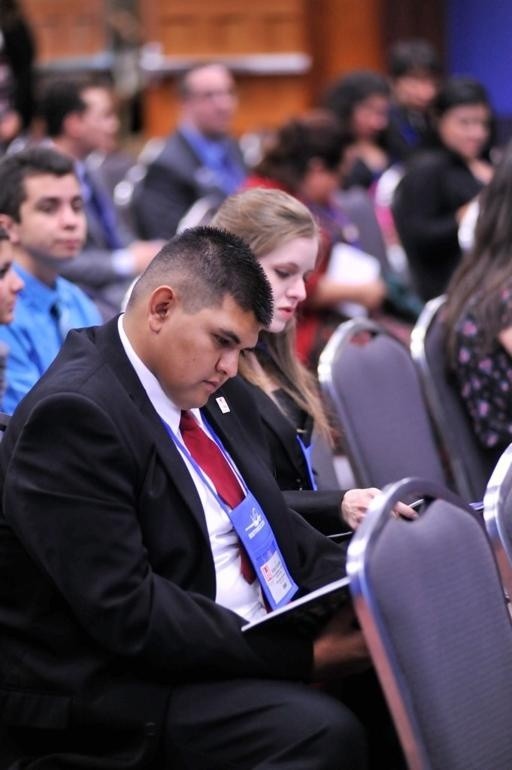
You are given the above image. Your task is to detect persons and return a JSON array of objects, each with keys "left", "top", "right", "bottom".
[
  {"left": 1, "top": 37, "right": 512, "bottom": 533},
  {"left": 0, "top": 224, "right": 370, "bottom": 770}
]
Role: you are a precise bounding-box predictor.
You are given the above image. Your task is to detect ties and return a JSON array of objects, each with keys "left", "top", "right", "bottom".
[
  {"left": 56, "top": 300, "right": 76, "bottom": 342},
  {"left": 180, "top": 411, "right": 259, "bottom": 584}
]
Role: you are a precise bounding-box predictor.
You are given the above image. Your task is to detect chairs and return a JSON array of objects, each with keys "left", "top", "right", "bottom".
[
  {"left": 409, "top": 295, "right": 493, "bottom": 523},
  {"left": 346, "top": 476, "right": 512, "bottom": 744},
  {"left": 482, "top": 443, "right": 512, "bottom": 568},
  {"left": 318, "top": 318, "right": 459, "bottom": 521}
]
